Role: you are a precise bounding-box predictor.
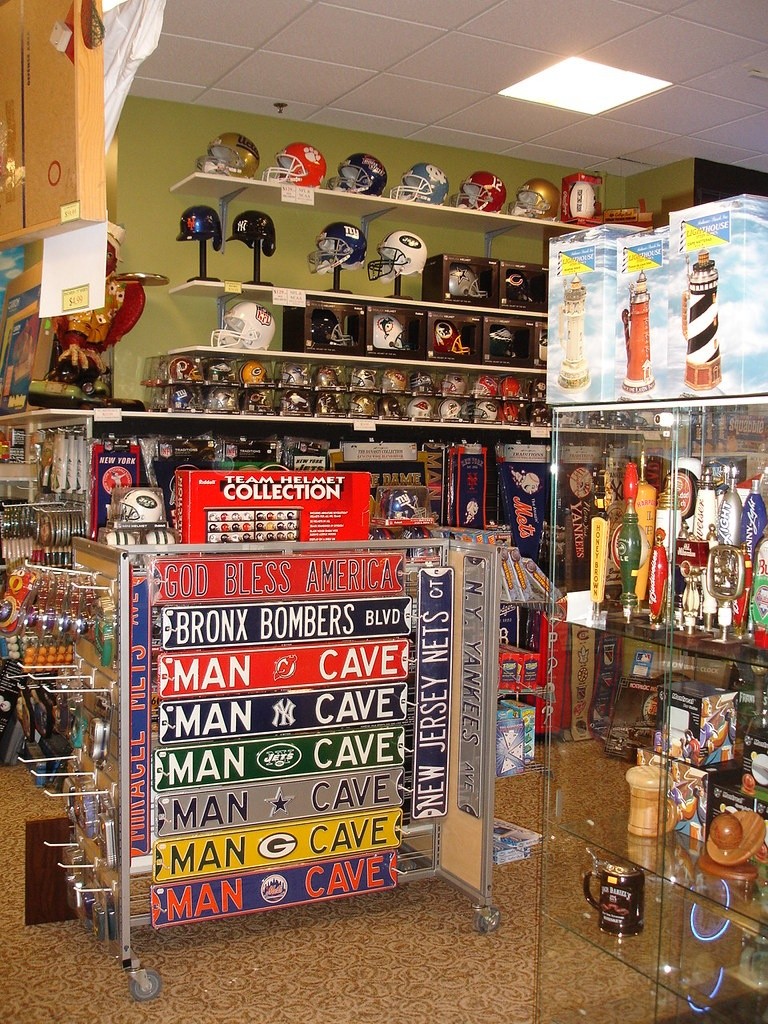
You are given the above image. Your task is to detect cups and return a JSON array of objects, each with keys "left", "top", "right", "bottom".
[
  {"left": 626, "top": 766, "right": 677, "bottom": 835},
  {"left": 584, "top": 862, "right": 647, "bottom": 936}
]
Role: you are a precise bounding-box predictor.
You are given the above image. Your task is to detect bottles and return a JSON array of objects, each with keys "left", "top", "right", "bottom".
[
  {"left": 693, "top": 467, "right": 718, "bottom": 540},
  {"left": 741, "top": 479, "right": 765, "bottom": 547},
  {"left": 717, "top": 478, "right": 743, "bottom": 549}
]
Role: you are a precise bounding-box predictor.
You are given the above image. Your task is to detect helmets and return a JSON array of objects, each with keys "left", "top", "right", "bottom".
[
  {"left": 312, "top": 309, "right": 340, "bottom": 344},
  {"left": 435, "top": 320, "right": 459, "bottom": 354},
  {"left": 460, "top": 171, "right": 506, "bottom": 212},
  {"left": 222, "top": 302, "right": 276, "bottom": 350},
  {"left": 517, "top": 178, "right": 560, "bottom": 219},
  {"left": 207, "top": 133, "right": 260, "bottom": 179},
  {"left": 316, "top": 222, "right": 367, "bottom": 271},
  {"left": 176, "top": 205, "right": 222, "bottom": 251},
  {"left": 490, "top": 326, "right": 513, "bottom": 356},
  {"left": 449, "top": 263, "right": 477, "bottom": 296},
  {"left": 338, "top": 153, "right": 387, "bottom": 197},
  {"left": 539, "top": 329, "right": 548, "bottom": 361},
  {"left": 169, "top": 357, "right": 552, "bottom": 427},
  {"left": 226, "top": 211, "right": 276, "bottom": 257},
  {"left": 505, "top": 269, "right": 528, "bottom": 300},
  {"left": 402, "top": 162, "right": 449, "bottom": 206},
  {"left": 373, "top": 314, "right": 405, "bottom": 350},
  {"left": 376, "top": 231, "right": 428, "bottom": 275},
  {"left": 275, "top": 143, "right": 327, "bottom": 188}
]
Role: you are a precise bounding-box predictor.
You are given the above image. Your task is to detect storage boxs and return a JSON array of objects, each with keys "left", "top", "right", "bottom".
[
  {"left": 172, "top": 470, "right": 372, "bottom": 542},
  {"left": 669, "top": 194, "right": 768, "bottom": 396},
  {"left": 422, "top": 255, "right": 498, "bottom": 307},
  {"left": 499, "top": 262, "right": 548, "bottom": 312},
  {"left": 496, "top": 700, "right": 536, "bottom": 776},
  {"left": 546, "top": 224, "right": 647, "bottom": 405},
  {"left": 499, "top": 644, "right": 540, "bottom": 690},
  {"left": 616, "top": 225, "right": 671, "bottom": 401}
]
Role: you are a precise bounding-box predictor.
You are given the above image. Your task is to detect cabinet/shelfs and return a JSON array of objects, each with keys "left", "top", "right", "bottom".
[
  {"left": 550, "top": 408, "right": 768, "bottom": 1024},
  {"left": 0, "top": 173, "right": 586, "bottom": 432}
]
